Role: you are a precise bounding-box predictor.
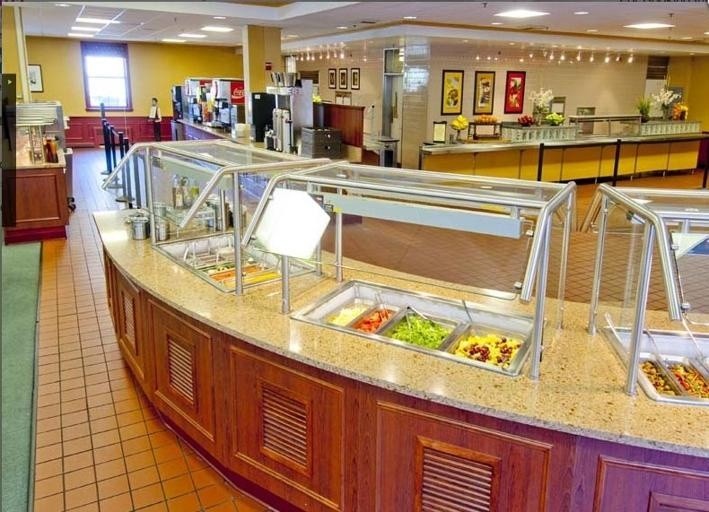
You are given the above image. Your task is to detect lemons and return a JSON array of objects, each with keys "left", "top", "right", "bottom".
[{"left": 451, "top": 115, "right": 468, "bottom": 131}]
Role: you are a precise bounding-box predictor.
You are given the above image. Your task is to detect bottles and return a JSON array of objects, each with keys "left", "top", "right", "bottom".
[
  {"left": 173, "top": 175, "right": 183, "bottom": 209},
  {"left": 43, "top": 139, "right": 58, "bottom": 163}
]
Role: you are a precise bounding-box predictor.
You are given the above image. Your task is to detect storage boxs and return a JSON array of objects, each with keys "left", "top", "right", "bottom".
[{"left": 301, "top": 126, "right": 344, "bottom": 159}]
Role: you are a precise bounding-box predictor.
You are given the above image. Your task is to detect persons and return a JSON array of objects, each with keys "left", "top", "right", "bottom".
[{"left": 147, "top": 97, "right": 162, "bottom": 141}]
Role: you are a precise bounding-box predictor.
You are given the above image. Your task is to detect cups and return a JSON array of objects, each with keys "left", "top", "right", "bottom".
[{"left": 130, "top": 193, "right": 247, "bottom": 241}]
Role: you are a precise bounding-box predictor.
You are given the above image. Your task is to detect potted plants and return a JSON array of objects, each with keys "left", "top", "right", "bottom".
[{"left": 637, "top": 94, "right": 653, "bottom": 122}]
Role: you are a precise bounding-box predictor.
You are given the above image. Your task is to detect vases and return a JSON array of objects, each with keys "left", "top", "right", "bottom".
[{"left": 663, "top": 106, "right": 671, "bottom": 121}]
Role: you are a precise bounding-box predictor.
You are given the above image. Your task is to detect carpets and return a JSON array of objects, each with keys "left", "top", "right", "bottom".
[{"left": 0, "top": 239, "right": 45, "bottom": 511}]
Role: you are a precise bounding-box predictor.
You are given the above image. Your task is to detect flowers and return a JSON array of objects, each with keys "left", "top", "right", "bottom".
[{"left": 653, "top": 88, "right": 681, "bottom": 105}]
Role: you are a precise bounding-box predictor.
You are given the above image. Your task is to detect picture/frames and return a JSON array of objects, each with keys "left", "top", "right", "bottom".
[
  {"left": 473, "top": 70, "right": 495, "bottom": 115},
  {"left": 328, "top": 67, "right": 360, "bottom": 90},
  {"left": 441, "top": 69, "right": 465, "bottom": 114},
  {"left": 504, "top": 71, "right": 526, "bottom": 113},
  {"left": 29, "top": 64, "right": 44, "bottom": 93}
]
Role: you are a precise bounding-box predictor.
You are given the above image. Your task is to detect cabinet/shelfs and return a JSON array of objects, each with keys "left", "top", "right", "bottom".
[
  {"left": 4, "top": 136, "right": 75, "bottom": 245},
  {"left": 316, "top": 102, "right": 364, "bottom": 148}
]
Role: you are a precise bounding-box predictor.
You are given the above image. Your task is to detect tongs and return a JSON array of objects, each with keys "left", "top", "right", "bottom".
[
  {"left": 373, "top": 293, "right": 390, "bottom": 322},
  {"left": 405, "top": 306, "right": 428, "bottom": 329}
]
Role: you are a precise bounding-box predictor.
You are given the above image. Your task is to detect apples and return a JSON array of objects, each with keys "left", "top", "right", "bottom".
[
  {"left": 545, "top": 113, "right": 565, "bottom": 124},
  {"left": 518, "top": 115, "right": 535, "bottom": 123}
]
